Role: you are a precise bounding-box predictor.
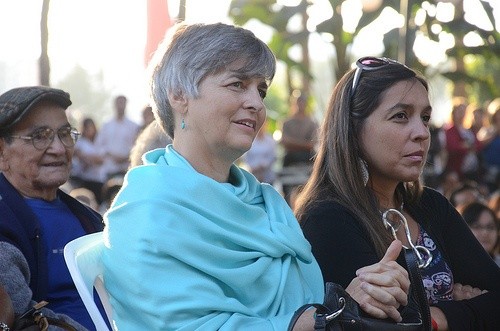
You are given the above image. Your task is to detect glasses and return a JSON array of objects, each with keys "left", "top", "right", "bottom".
[
  {"left": 10, "top": 126, "right": 80, "bottom": 151},
  {"left": 349, "top": 57, "right": 413, "bottom": 99}
]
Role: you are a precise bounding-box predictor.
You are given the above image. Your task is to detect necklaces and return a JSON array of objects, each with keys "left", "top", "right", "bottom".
[{"left": 366, "top": 185, "right": 405, "bottom": 232}]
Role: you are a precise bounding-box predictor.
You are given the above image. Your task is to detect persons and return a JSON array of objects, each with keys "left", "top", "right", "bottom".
[
  {"left": 0, "top": 86, "right": 500, "bottom": 331},
  {"left": 293, "top": 57, "right": 500, "bottom": 331},
  {"left": 97, "top": 22, "right": 326, "bottom": 331}
]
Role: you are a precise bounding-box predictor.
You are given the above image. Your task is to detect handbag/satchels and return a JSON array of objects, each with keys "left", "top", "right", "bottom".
[
  {"left": 285, "top": 249, "right": 432, "bottom": 331},
  {"left": 0, "top": 301, "right": 78, "bottom": 331}
]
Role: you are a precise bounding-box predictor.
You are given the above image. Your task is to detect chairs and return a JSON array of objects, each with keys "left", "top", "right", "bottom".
[{"left": 64, "top": 232, "right": 120, "bottom": 331}]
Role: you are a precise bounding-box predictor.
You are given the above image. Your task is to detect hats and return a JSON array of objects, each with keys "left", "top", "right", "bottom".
[{"left": 0, "top": 86, "right": 72, "bottom": 128}]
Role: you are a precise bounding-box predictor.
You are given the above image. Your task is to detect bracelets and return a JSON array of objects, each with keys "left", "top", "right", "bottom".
[{"left": 431, "top": 317, "right": 438, "bottom": 331}]
